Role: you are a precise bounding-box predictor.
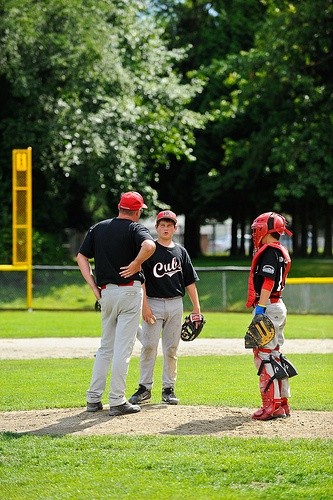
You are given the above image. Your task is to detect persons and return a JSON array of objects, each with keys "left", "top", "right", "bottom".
[
  {"left": 77, "top": 191, "right": 157, "bottom": 416},
  {"left": 246, "top": 212, "right": 292, "bottom": 421},
  {"left": 129, "top": 210, "right": 201, "bottom": 404}
]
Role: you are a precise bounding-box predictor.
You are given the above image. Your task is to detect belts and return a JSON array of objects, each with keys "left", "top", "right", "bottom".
[
  {"left": 269, "top": 296, "right": 282, "bottom": 304},
  {"left": 101, "top": 280, "right": 134, "bottom": 288}
]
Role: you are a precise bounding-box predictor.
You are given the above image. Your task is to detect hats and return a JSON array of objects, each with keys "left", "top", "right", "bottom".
[
  {"left": 156, "top": 210, "right": 178, "bottom": 225},
  {"left": 118, "top": 192, "right": 148, "bottom": 210}
]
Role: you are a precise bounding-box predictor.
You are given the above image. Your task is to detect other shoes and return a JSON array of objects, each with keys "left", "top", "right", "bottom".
[{"left": 252, "top": 403, "right": 291, "bottom": 420}]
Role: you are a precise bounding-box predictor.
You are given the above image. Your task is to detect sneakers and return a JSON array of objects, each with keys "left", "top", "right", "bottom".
[
  {"left": 162, "top": 388, "right": 179, "bottom": 405},
  {"left": 109, "top": 400, "right": 141, "bottom": 416},
  {"left": 128, "top": 384, "right": 151, "bottom": 404},
  {"left": 87, "top": 402, "right": 103, "bottom": 412}
]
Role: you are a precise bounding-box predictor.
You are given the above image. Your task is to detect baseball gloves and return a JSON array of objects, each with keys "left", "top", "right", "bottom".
[
  {"left": 244, "top": 314, "right": 275, "bottom": 348},
  {"left": 181, "top": 313, "right": 205, "bottom": 342}
]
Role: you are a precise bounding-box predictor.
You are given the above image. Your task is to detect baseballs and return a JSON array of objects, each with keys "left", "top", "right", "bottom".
[{"left": 150, "top": 315, "right": 156, "bottom": 324}]
